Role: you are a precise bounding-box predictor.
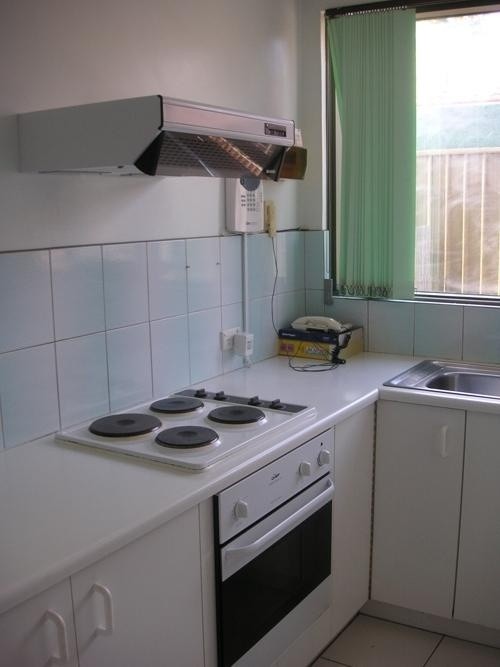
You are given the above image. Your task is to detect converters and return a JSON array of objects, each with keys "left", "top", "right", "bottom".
[{"left": 233, "top": 333, "right": 253, "bottom": 357}]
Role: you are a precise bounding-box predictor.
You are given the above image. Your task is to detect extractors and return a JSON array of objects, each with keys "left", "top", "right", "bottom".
[{"left": 16, "top": 95, "right": 306, "bottom": 180}]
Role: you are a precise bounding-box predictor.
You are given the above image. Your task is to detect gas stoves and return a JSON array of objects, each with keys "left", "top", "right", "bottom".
[{"left": 56, "top": 389, "right": 319, "bottom": 472}]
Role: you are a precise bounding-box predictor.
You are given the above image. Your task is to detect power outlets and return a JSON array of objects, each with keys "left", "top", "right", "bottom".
[{"left": 220, "top": 326, "right": 244, "bottom": 352}]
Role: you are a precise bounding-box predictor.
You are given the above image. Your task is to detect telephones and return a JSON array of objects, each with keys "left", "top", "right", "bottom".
[{"left": 292, "top": 316, "right": 352, "bottom": 333}]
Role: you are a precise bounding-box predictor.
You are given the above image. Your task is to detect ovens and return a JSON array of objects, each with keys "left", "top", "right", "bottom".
[{"left": 211, "top": 421, "right": 335, "bottom": 667}]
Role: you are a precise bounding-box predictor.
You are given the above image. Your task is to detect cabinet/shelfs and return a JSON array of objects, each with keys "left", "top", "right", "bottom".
[
  {"left": 371, "top": 384, "right": 500, "bottom": 647},
  {"left": 332, "top": 402, "right": 377, "bottom": 636},
  {"left": 0, "top": 494, "right": 220, "bottom": 667}
]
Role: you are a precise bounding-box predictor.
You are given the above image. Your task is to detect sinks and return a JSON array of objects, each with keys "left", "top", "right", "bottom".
[{"left": 385, "top": 357, "right": 499, "bottom": 405}]
